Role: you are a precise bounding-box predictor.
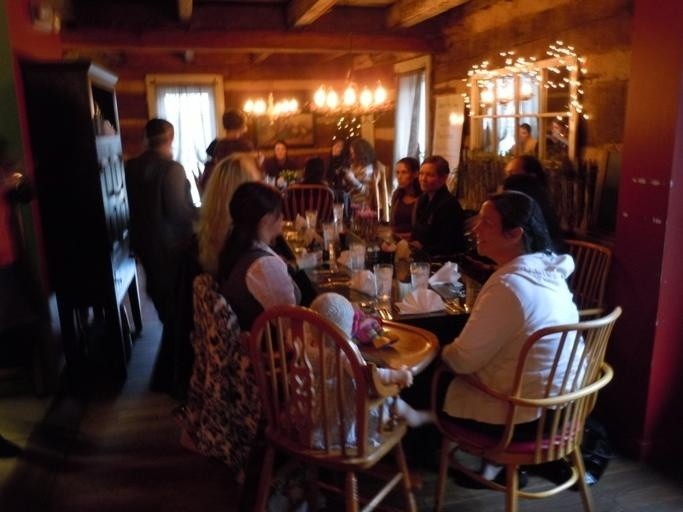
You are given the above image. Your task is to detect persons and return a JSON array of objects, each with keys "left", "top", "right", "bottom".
[
  {"left": 544, "top": 117, "right": 569, "bottom": 156},
  {"left": 510, "top": 124, "right": 540, "bottom": 160},
  {"left": 504, "top": 155, "right": 558, "bottom": 246},
  {"left": 443, "top": 190, "right": 589, "bottom": 490},
  {"left": 212, "top": 183, "right": 316, "bottom": 333},
  {"left": 496, "top": 174, "right": 562, "bottom": 254},
  {"left": 325, "top": 138, "right": 350, "bottom": 182},
  {"left": 201, "top": 107, "right": 258, "bottom": 183},
  {"left": 350, "top": 139, "right": 389, "bottom": 224},
  {"left": 299, "top": 293, "right": 437, "bottom": 448},
  {"left": 128, "top": 119, "right": 199, "bottom": 401},
  {"left": 301, "top": 156, "right": 327, "bottom": 185},
  {"left": 390, "top": 156, "right": 422, "bottom": 230},
  {"left": 411, "top": 155, "right": 465, "bottom": 255},
  {"left": 200, "top": 153, "right": 259, "bottom": 284},
  {"left": 259, "top": 139, "right": 296, "bottom": 176}
]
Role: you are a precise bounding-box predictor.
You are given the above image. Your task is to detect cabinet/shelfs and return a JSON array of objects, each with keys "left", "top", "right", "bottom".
[{"left": 23, "top": 59, "right": 142, "bottom": 392}]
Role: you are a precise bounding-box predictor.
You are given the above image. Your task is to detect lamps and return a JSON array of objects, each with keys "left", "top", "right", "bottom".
[
  {"left": 307, "top": 19, "right": 392, "bottom": 116},
  {"left": 243, "top": 80, "right": 301, "bottom": 126}
]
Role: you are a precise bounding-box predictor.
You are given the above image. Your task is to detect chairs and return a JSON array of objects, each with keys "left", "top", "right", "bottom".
[
  {"left": 557, "top": 239, "right": 611, "bottom": 317},
  {"left": 277, "top": 182, "right": 334, "bottom": 220},
  {"left": 239, "top": 304, "right": 439, "bottom": 512},
  {"left": 185, "top": 273, "right": 251, "bottom": 482},
  {"left": 429, "top": 306, "right": 623, "bottom": 512}
]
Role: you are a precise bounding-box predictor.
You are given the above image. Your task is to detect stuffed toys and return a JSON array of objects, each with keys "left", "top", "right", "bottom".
[{"left": 350, "top": 309, "right": 400, "bottom": 348}]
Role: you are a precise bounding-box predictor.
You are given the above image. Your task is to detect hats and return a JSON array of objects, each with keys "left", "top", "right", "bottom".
[{"left": 307, "top": 292, "right": 352, "bottom": 339}]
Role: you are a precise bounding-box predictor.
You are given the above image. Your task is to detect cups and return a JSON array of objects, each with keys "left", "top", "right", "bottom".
[
  {"left": 332, "top": 202, "right": 344, "bottom": 225},
  {"left": 349, "top": 241, "right": 366, "bottom": 272},
  {"left": 305, "top": 209, "right": 317, "bottom": 230},
  {"left": 373, "top": 264, "right": 394, "bottom": 299},
  {"left": 322, "top": 222, "right": 337, "bottom": 257},
  {"left": 409, "top": 262, "right": 431, "bottom": 289}
]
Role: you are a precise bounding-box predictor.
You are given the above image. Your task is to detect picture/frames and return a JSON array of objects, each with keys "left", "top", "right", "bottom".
[{"left": 253, "top": 113, "right": 316, "bottom": 148}]
